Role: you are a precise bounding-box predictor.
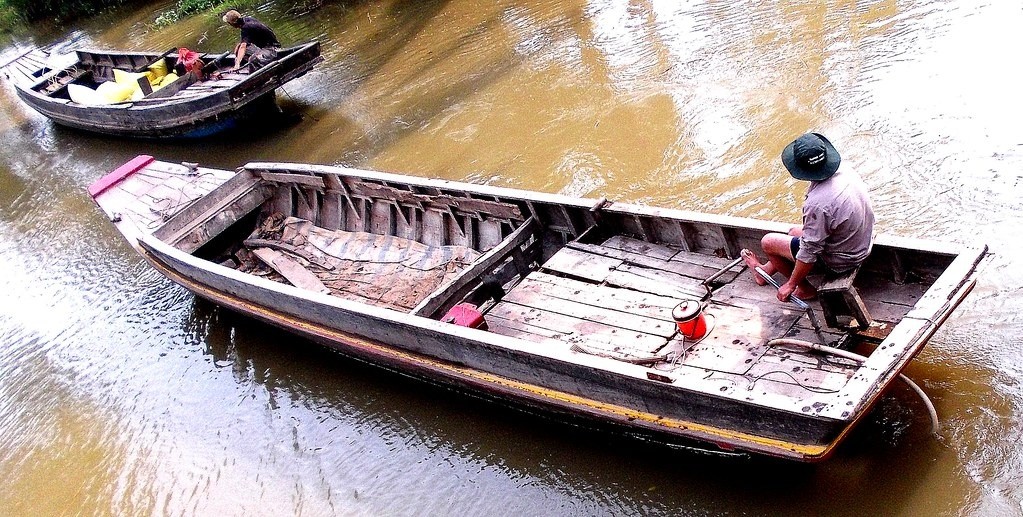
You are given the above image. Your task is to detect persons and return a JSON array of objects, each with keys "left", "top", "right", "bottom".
[
  {"left": 223, "top": 10, "right": 278, "bottom": 74},
  {"left": 741, "top": 133, "right": 875, "bottom": 302}
]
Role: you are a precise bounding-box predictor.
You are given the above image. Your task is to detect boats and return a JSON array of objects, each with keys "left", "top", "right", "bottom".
[
  {"left": 0, "top": 39, "right": 326, "bottom": 147},
  {"left": 85, "top": 151, "right": 990, "bottom": 466}
]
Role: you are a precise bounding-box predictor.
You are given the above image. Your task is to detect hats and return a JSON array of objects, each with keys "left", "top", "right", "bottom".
[{"left": 782, "top": 132, "right": 841, "bottom": 181}]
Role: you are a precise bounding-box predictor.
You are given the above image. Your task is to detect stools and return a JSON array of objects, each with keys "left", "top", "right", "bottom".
[{"left": 817, "top": 266, "right": 871, "bottom": 333}]
